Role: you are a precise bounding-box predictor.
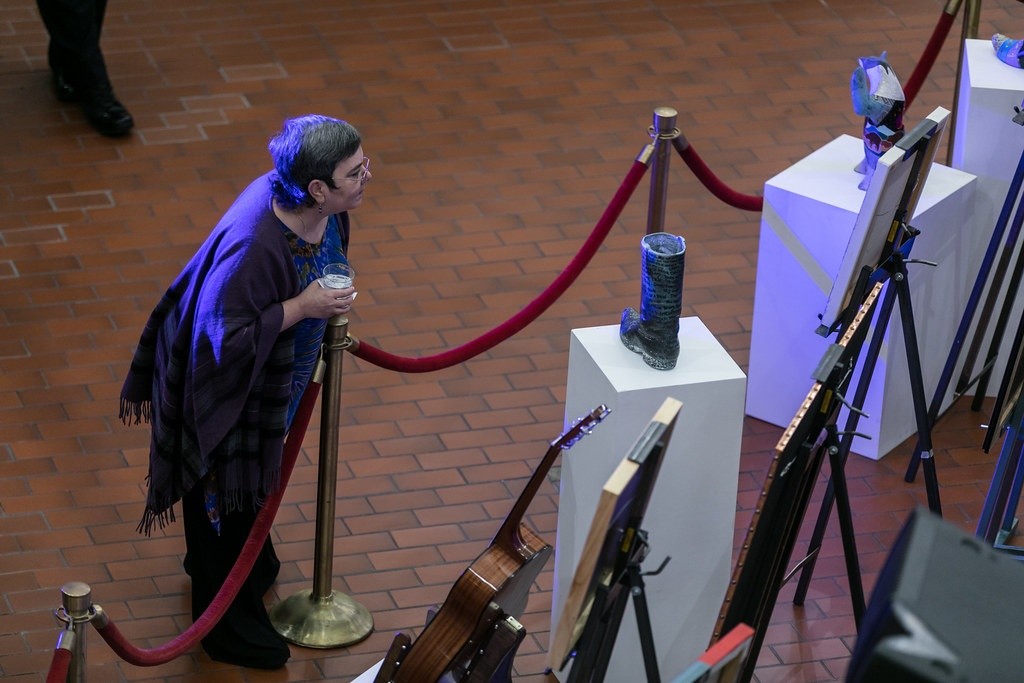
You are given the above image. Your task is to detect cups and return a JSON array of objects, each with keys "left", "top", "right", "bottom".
[{"left": 321, "top": 262, "right": 355, "bottom": 290}]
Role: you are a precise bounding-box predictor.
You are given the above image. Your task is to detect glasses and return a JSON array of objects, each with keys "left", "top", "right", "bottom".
[{"left": 331, "top": 156, "right": 371, "bottom": 182}]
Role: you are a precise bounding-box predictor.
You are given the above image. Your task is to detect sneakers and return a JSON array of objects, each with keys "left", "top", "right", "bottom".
[{"left": 48, "top": 43, "right": 134, "bottom": 137}]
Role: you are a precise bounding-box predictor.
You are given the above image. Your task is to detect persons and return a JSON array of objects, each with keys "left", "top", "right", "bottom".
[
  {"left": 33, "top": 0, "right": 134, "bottom": 141},
  {"left": 119, "top": 111, "right": 373, "bottom": 670}
]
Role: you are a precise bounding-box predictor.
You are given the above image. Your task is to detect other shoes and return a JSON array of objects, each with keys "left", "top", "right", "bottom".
[{"left": 201, "top": 623, "right": 290, "bottom": 670}]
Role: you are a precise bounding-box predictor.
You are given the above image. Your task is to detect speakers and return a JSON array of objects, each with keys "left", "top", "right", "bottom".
[{"left": 843, "top": 505, "right": 1024, "bottom": 683}]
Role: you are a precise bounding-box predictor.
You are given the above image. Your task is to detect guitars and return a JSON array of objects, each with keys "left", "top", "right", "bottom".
[{"left": 372, "top": 402, "right": 614, "bottom": 683}]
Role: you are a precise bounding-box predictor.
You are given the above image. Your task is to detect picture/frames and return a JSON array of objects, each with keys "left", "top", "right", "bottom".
[
  {"left": 546, "top": 397, "right": 683, "bottom": 672},
  {"left": 671, "top": 623, "right": 755, "bottom": 683},
  {"left": 706, "top": 282, "right": 883, "bottom": 683},
  {"left": 821, "top": 106, "right": 952, "bottom": 328}
]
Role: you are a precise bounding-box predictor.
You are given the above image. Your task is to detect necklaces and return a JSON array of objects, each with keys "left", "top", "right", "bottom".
[{"left": 297, "top": 214, "right": 331, "bottom": 277}]
